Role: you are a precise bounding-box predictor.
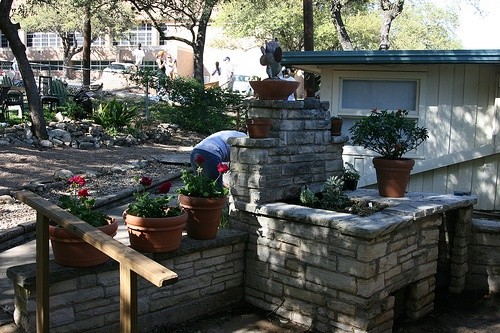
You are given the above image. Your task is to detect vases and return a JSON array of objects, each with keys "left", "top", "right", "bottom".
[
  {"left": 123, "top": 207, "right": 187, "bottom": 252},
  {"left": 177, "top": 194, "right": 227, "bottom": 240},
  {"left": 47, "top": 215, "right": 118, "bottom": 266}
]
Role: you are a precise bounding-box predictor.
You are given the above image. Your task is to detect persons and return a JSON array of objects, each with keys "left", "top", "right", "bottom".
[
  {"left": 156, "top": 49, "right": 169, "bottom": 73},
  {"left": 132, "top": 43, "right": 147, "bottom": 66},
  {"left": 220, "top": 56, "right": 234, "bottom": 92},
  {"left": 280, "top": 67, "right": 296, "bottom": 101},
  {"left": 191, "top": 129, "right": 250, "bottom": 192},
  {"left": 165, "top": 54, "right": 175, "bottom": 79}
]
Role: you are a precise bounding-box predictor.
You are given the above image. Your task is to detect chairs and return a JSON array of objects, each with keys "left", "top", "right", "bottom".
[
  {"left": 3, "top": 88, "right": 25, "bottom": 122},
  {"left": 38, "top": 75, "right": 59, "bottom": 113}
]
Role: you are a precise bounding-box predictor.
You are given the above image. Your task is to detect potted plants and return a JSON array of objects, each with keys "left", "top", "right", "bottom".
[
  {"left": 0, "top": 74, "right": 11, "bottom": 95},
  {"left": 330, "top": 114, "right": 345, "bottom": 138},
  {"left": 349, "top": 108, "right": 430, "bottom": 198},
  {"left": 246, "top": 115, "right": 274, "bottom": 138},
  {"left": 341, "top": 162, "right": 359, "bottom": 191},
  {"left": 249, "top": 40, "right": 301, "bottom": 101}
]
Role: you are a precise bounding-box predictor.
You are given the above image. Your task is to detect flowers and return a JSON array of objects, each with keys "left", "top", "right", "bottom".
[
  {"left": 128, "top": 173, "right": 182, "bottom": 216},
  {"left": 181, "top": 155, "right": 231, "bottom": 199},
  {"left": 56, "top": 174, "right": 107, "bottom": 228}
]
